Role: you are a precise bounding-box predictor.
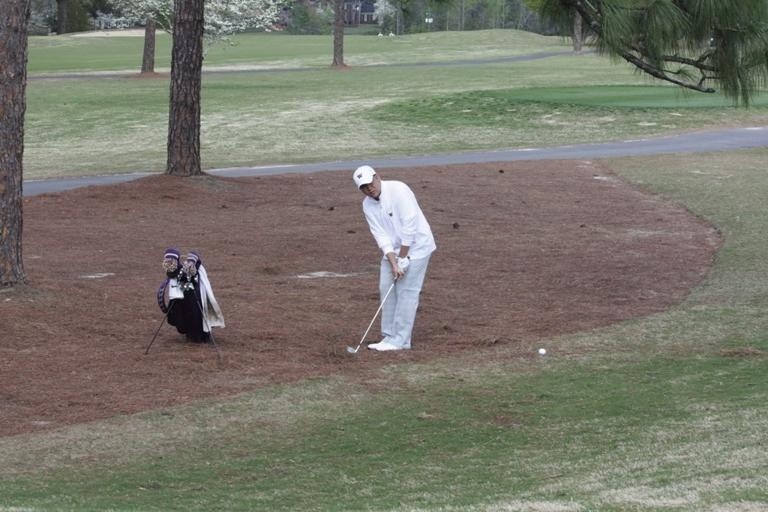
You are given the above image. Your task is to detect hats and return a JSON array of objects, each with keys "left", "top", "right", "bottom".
[{"left": 353, "top": 165, "right": 377, "bottom": 192}]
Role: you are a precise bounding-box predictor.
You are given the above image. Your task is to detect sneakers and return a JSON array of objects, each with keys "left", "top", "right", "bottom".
[{"left": 367, "top": 336, "right": 411, "bottom": 352}]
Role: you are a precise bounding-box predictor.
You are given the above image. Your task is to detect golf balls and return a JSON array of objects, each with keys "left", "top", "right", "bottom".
[
  {"left": 145, "top": 247, "right": 222, "bottom": 361},
  {"left": 539, "top": 348, "right": 546, "bottom": 355}
]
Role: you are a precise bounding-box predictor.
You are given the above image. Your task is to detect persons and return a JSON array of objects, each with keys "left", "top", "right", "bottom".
[{"left": 352, "top": 163, "right": 439, "bottom": 353}]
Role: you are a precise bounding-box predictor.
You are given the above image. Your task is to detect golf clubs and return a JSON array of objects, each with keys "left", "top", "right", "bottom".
[{"left": 346, "top": 256, "right": 410, "bottom": 353}]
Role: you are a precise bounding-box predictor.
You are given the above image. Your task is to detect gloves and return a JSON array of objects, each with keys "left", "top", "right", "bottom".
[{"left": 396, "top": 256, "right": 410, "bottom": 272}]
